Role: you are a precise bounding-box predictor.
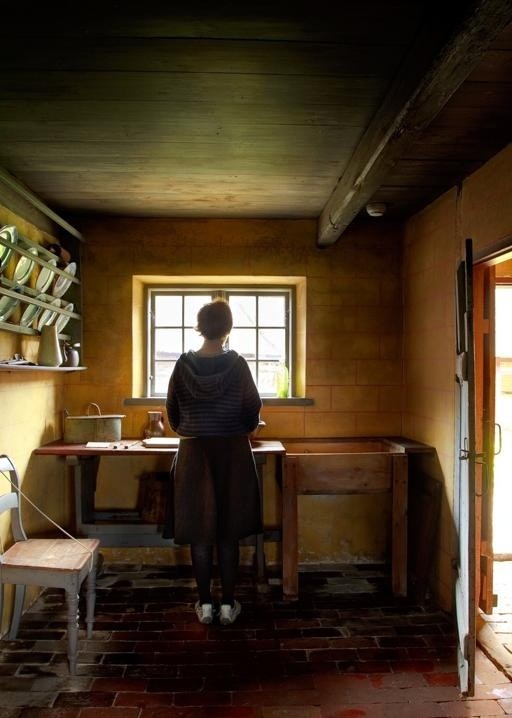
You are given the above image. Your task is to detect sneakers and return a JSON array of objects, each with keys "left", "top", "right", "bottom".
[
  {"left": 218, "top": 599, "right": 242, "bottom": 625},
  {"left": 194, "top": 599, "right": 214, "bottom": 624}
]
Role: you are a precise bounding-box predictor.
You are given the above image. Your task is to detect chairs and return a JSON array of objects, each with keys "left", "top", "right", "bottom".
[{"left": 0, "top": 451, "right": 100, "bottom": 678}]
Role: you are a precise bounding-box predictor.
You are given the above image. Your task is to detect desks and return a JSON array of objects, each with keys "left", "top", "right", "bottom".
[{"left": 35, "top": 438, "right": 285, "bottom": 535}]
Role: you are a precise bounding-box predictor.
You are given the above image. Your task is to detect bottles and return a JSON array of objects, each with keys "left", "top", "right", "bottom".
[
  {"left": 36, "top": 324, "right": 63, "bottom": 366},
  {"left": 143, "top": 411, "right": 166, "bottom": 439}
]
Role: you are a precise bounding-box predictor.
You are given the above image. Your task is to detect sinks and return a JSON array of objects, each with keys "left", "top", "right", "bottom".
[{"left": 142, "top": 437, "right": 179, "bottom": 448}]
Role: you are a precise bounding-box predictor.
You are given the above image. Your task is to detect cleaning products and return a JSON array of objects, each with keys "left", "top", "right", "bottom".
[{"left": 275, "top": 361, "right": 289, "bottom": 398}]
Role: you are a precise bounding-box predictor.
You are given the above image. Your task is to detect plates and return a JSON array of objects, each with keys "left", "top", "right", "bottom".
[
  {"left": 53, "top": 261, "right": 77, "bottom": 298},
  {"left": 53, "top": 303, "right": 74, "bottom": 333},
  {"left": 0, "top": 224, "right": 19, "bottom": 275},
  {"left": 13, "top": 247, "right": 39, "bottom": 284},
  {"left": 20, "top": 293, "right": 48, "bottom": 328},
  {"left": 35, "top": 258, "right": 57, "bottom": 293},
  {"left": 0, "top": 285, "right": 25, "bottom": 322},
  {"left": 37, "top": 299, "right": 62, "bottom": 332}
]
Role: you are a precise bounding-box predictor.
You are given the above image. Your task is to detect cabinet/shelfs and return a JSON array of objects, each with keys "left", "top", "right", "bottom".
[{"left": 0, "top": 171, "right": 89, "bottom": 375}]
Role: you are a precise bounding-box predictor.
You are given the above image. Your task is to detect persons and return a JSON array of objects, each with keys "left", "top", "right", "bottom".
[{"left": 166, "top": 300, "right": 264, "bottom": 624}]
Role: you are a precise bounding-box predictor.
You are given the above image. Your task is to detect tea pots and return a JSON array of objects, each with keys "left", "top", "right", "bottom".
[{"left": 59, "top": 341, "right": 80, "bottom": 367}]
